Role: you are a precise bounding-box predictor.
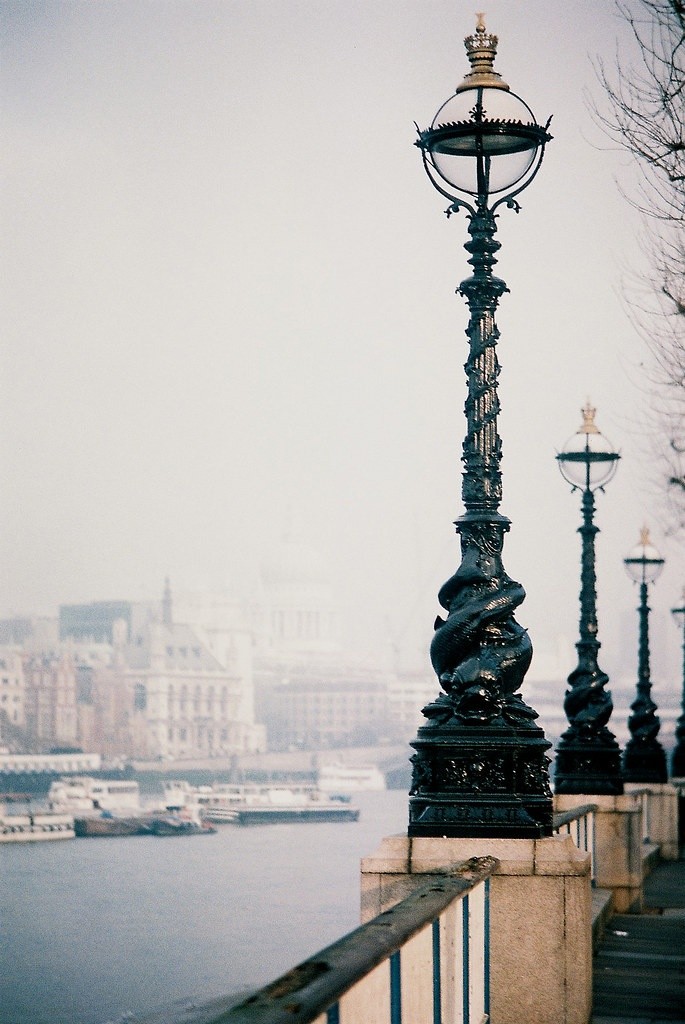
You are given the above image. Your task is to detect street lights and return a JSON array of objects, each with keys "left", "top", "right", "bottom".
[
  {"left": 548, "top": 403, "right": 630, "bottom": 799},
  {"left": 621, "top": 525, "right": 672, "bottom": 786},
  {"left": 670, "top": 584, "right": 685, "bottom": 781},
  {"left": 377, "top": 11, "right": 565, "bottom": 839}
]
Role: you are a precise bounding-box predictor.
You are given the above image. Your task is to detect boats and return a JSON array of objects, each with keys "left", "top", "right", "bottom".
[
  {"left": 0, "top": 793, "right": 75, "bottom": 844},
  {"left": 48, "top": 768, "right": 365, "bottom": 844}
]
